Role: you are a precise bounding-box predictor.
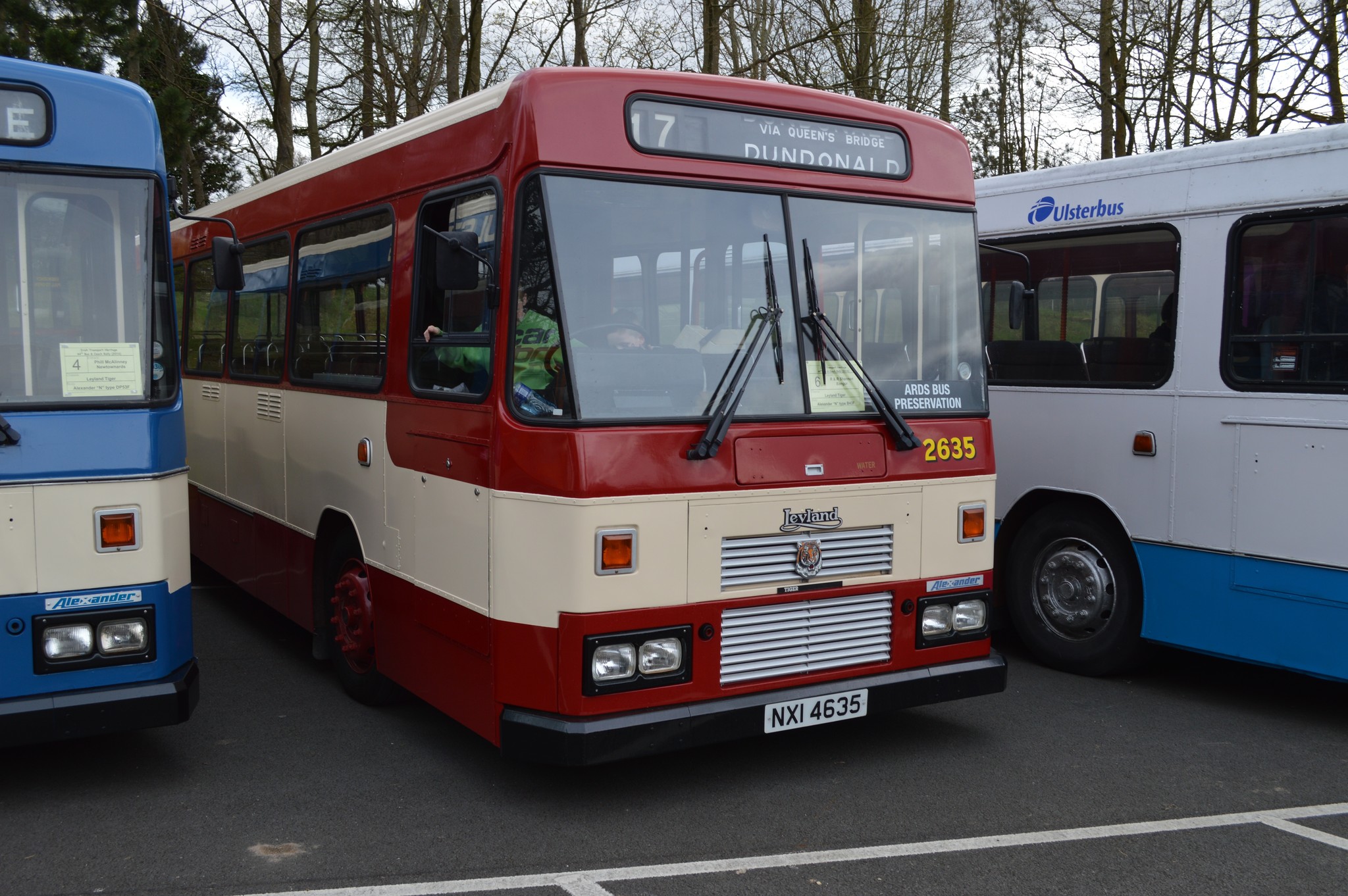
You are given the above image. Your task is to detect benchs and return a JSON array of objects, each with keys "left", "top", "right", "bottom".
[{"left": 179, "top": 330, "right": 386, "bottom": 376}]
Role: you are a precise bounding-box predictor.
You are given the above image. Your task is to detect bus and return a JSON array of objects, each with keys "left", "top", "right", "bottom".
[
  {"left": 170, "top": 66, "right": 1010, "bottom": 772},
  {"left": 615, "top": 121, "right": 1348, "bottom": 686},
  {"left": 0, "top": 52, "right": 253, "bottom": 716}
]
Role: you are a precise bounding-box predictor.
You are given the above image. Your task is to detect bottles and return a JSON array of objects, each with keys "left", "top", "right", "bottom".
[{"left": 513, "top": 381, "right": 557, "bottom": 415}]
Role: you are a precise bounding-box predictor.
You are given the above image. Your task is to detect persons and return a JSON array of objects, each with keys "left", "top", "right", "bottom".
[{"left": 423, "top": 263, "right": 595, "bottom": 400}]
